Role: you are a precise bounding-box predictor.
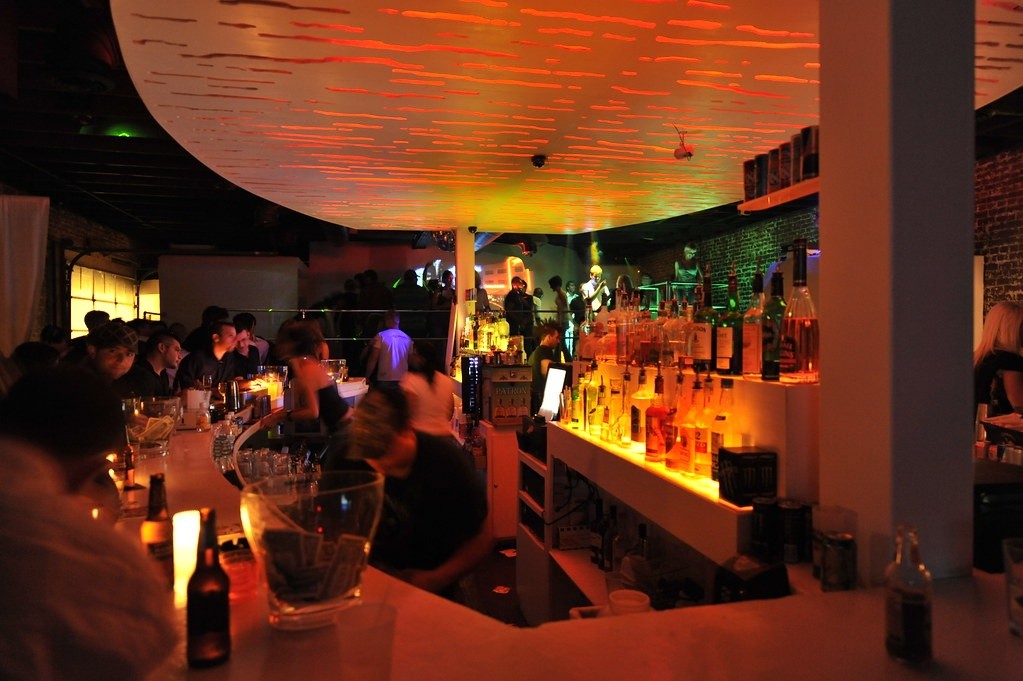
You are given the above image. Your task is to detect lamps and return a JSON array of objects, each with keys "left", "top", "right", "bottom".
[
  {"left": 517, "top": 240, "right": 537, "bottom": 257},
  {"left": 410, "top": 231, "right": 431, "bottom": 250},
  {"left": 674, "top": 130, "right": 695, "bottom": 163},
  {"left": 531, "top": 155, "right": 546, "bottom": 168}
]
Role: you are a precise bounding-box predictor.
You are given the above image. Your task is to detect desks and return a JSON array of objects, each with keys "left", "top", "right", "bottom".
[{"left": 975, "top": 459, "right": 1023, "bottom": 482}]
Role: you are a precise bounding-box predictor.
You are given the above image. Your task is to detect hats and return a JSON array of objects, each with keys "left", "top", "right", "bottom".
[
  {"left": 345, "top": 387, "right": 408, "bottom": 459},
  {"left": 85, "top": 322, "right": 139, "bottom": 354}
]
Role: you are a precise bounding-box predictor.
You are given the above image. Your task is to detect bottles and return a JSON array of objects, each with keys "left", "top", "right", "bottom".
[
  {"left": 553, "top": 238, "right": 818, "bottom": 486},
  {"left": 585, "top": 499, "right": 707, "bottom": 614},
  {"left": 192, "top": 373, "right": 325, "bottom": 540},
  {"left": 140, "top": 473, "right": 177, "bottom": 597},
  {"left": 187, "top": 507, "right": 232, "bottom": 669},
  {"left": 884, "top": 524, "right": 935, "bottom": 666},
  {"left": 460, "top": 306, "right": 528, "bottom": 366}
]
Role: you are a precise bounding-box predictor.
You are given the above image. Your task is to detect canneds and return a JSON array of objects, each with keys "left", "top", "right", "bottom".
[
  {"left": 750, "top": 495, "right": 858, "bottom": 591},
  {"left": 259, "top": 395, "right": 271, "bottom": 417}
]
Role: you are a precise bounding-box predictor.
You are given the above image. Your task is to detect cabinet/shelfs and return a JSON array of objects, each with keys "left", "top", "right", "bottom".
[{"left": 513, "top": 363, "right": 819, "bottom": 625}]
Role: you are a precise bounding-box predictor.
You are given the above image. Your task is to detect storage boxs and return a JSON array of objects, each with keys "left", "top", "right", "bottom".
[
  {"left": 556, "top": 524, "right": 592, "bottom": 551},
  {"left": 980, "top": 412, "right": 1023, "bottom": 457},
  {"left": 719, "top": 447, "right": 778, "bottom": 508}
]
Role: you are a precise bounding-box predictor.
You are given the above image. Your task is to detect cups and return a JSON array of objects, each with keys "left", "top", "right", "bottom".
[
  {"left": 241, "top": 470, "right": 385, "bottom": 631},
  {"left": 107, "top": 392, "right": 188, "bottom": 471},
  {"left": 334, "top": 601, "right": 396, "bottom": 680},
  {"left": 322, "top": 359, "right": 346, "bottom": 383},
  {"left": 257, "top": 365, "right": 288, "bottom": 388}
]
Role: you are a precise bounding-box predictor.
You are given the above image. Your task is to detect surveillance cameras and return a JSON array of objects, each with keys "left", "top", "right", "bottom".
[
  {"left": 468, "top": 226, "right": 477, "bottom": 233},
  {"left": 530, "top": 155, "right": 547, "bottom": 167}
]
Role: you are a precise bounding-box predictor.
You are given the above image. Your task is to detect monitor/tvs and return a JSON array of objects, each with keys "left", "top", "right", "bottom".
[{"left": 539, "top": 363, "right": 571, "bottom": 421}]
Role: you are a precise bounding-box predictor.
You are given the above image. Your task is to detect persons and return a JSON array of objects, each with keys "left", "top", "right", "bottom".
[
  {"left": 581, "top": 265, "right": 611, "bottom": 335},
  {"left": 273, "top": 320, "right": 333, "bottom": 461},
  {"left": 530, "top": 324, "right": 572, "bottom": 417},
  {"left": 399, "top": 339, "right": 454, "bottom": 442},
  {"left": 365, "top": 311, "right": 412, "bottom": 381},
  {"left": 504, "top": 276, "right": 588, "bottom": 363},
  {"left": 476, "top": 270, "right": 490, "bottom": 310},
  {"left": 596, "top": 274, "right": 663, "bottom": 316},
  {"left": 669, "top": 243, "right": 704, "bottom": 305},
  {"left": 0, "top": 366, "right": 179, "bottom": 681},
  {"left": 973, "top": 300, "right": 1023, "bottom": 574},
  {"left": 0, "top": 306, "right": 273, "bottom": 403},
  {"left": 320, "top": 382, "right": 496, "bottom": 594},
  {"left": 294, "top": 268, "right": 457, "bottom": 377}
]
